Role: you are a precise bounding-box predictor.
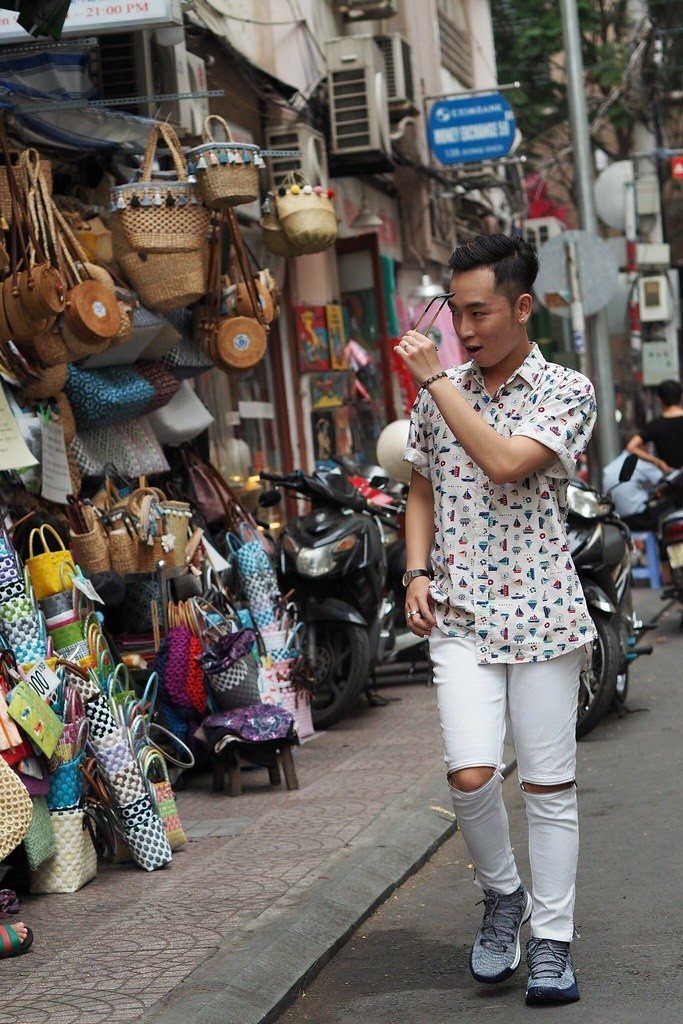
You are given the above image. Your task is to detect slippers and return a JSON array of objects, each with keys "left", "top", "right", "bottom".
[{"left": 0, "top": 924, "right": 32, "bottom": 959}]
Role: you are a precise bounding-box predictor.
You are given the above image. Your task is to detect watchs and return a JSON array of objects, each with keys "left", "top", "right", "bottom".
[{"left": 401, "top": 569, "right": 429, "bottom": 589}]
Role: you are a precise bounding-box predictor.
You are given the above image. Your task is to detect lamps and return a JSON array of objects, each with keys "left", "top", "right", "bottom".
[{"left": 349, "top": 181, "right": 384, "bottom": 227}]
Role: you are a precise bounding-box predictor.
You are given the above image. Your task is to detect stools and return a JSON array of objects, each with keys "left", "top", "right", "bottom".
[
  {"left": 210, "top": 730, "right": 301, "bottom": 796},
  {"left": 629, "top": 531, "right": 662, "bottom": 589}
]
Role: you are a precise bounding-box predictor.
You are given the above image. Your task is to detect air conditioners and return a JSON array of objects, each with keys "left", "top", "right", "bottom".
[
  {"left": 264, "top": 123, "right": 327, "bottom": 194},
  {"left": 373, "top": 32, "right": 414, "bottom": 107},
  {"left": 327, "top": 34, "right": 397, "bottom": 174},
  {"left": 186, "top": 50, "right": 212, "bottom": 135}
]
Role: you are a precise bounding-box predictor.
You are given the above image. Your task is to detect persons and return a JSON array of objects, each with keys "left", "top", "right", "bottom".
[
  {"left": 601, "top": 429, "right": 673, "bottom": 584},
  {"left": 400, "top": 231, "right": 597, "bottom": 1002},
  {"left": 626, "top": 380, "right": 683, "bottom": 477},
  {"left": 613, "top": 383, "right": 661, "bottom": 444}
]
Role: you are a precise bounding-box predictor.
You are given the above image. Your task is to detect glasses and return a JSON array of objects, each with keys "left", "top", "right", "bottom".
[{"left": 411, "top": 292, "right": 455, "bottom": 351}]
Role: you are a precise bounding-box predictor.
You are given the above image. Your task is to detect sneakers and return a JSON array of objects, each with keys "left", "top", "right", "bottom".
[
  {"left": 525, "top": 938, "right": 581, "bottom": 1006},
  {"left": 468, "top": 883, "right": 532, "bottom": 986}
]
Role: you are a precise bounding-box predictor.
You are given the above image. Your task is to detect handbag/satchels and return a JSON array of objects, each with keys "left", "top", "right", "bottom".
[
  {"left": 0, "top": 124, "right": 337, "bottom": 893},
  {"left": 187, "top": 114, "right": 260, "bottom": 210}
]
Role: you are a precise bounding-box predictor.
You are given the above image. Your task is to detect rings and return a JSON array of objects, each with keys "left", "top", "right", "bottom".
[{"left": 407, "top": 610, "right": 420, "bottom": 618}]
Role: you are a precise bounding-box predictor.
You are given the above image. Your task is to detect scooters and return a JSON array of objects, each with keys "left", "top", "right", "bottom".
[
  {"left": 562, "top": 453, "right": 662, "bottom": 743},
  {"left": 254, "top": 418, "right": 434, "bottom": 732},
  {"left": 639, "top": 464, "right": 683, "bottom": 633}
]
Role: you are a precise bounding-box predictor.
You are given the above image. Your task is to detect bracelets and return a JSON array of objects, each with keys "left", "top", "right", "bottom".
[{"left": 420, "top": 371, "right": 448, "bottom": 389}]
